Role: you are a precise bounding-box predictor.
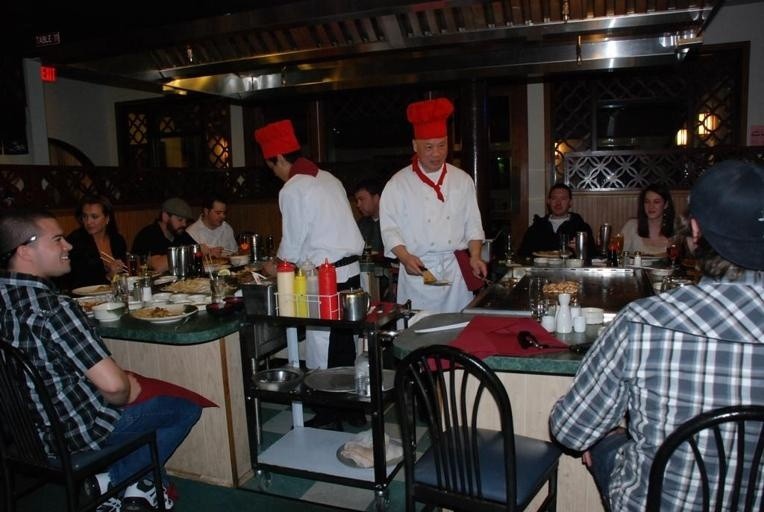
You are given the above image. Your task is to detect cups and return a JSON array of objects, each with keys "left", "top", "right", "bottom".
[
  {"left": 614, "top": 233, "right": 624, "bottom": 259},
  {"left": 529, "top": 277, "right": 548, "bottom": 322},
  {"left": 560, "top": 233, "right": 569, "bottom": 258},
  {"left": 574, "top": 316, "right": 586, "bottom": 332},
  {"left": 607, "top": 239, "right": 617, "bottom": 267},
  {"left": 541, "top": 316, "right": 555, "bottom": 333}
]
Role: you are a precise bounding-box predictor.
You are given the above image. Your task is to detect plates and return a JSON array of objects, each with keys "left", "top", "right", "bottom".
[
  {"left": 72, "top": 285, "right": 115, "bottom": 295},
  {"left": 129, "top": 303, "right": 198, "bottom": 324},
  {"left": 531, "top": 251, "right": 572, "bottom": 258}
]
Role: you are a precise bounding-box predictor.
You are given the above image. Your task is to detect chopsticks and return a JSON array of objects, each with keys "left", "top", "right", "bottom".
[{"left": 100, "top": 251, "right": 128, "bottom": 273}]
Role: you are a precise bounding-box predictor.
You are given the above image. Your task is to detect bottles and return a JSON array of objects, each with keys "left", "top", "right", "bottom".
[
  {"left": 141, "top": 278, "right": 151, "bottom": 302},
  {"left": 571, "top": 298, "right": 581, "bottom": 327},
  {"left": 300, "top": 257, "right": 314, "bottom": 273},
  {"left": 607, "top": 243, "right": 618, "bottom": 267},
  {"left": 634, "top": 251, "right": 641, "bottom": 266},
  {"left": 307, "top": 269, "right": 319, "bottom": 319},
  {"left": 318, "top": 258, "right": 337, "bottom": 320},
  {"left": 133, "top": 282, "right": 141, "bottom": 301},
  {"left": 294, "top": 268, "right": 309, "bottom": 318},
  {"left": 555, "top": 294, "right": 571, "bottom": 334},
  {"left": 622, "top": 251, "right": 629, "bottom": 268},
  {"left": 277, "top": 257, "right": 295, "bottom": 317},
  {"left": 504, "top": 235, "right": 514, "bottom": 264},
  {"left": 191, "top": 252, "right": 205, "bottom": 280},
  {"left": 130, "top": 255, "right": 136, "bottom": 275},
  {"left": 354, "top": 336, "right": 371, "bottom": 398}
]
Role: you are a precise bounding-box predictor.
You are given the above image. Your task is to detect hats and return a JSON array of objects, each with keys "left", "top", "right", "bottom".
[
  {"left": 161, "top": 198, "right": 194, "bottom": 219},
  {"left": 688, "top": 159, "right": 763, "bottom": 272},
  {"left": 406, "top": 96, "right": 455, "bottom": 139},
  {"left": 254, "top": 118, "right": 300, "bottom": 160}
]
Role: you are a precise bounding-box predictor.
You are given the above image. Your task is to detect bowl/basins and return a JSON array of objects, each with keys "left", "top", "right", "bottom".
[
  {"left": 250, "top": 367, "right": 304, "bottom": 393},
  {"left": 229, "top": 256, "right": 248, "bottom": 266},
  {"left": 91, "top": 302, "right": 125, "bottom": 323},
  {"left": 581, "top": 308, "right": 605, "bottom": 325}
]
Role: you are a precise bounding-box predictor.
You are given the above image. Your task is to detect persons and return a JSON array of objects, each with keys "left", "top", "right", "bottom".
[
  {"left": 618, "top": 183, "right": 679, "bottom": 256},
  {"left": 378, "top": 97, "right": 487, "bottom": 313},
  {"left": 184, "top": 193, "right": 239, "bottom": 254},
  {"left": 0, "top": 204, "right": 202, "bottom": 512},
  {"left": 353, "top": 177, "right": 386, "bottom": 250},
  {"left": 129, "top": 197, "right": 212, "bottom": 273},
  {"left": 517, "top": 182, "right": 596, "bottom": 261},
  {"left": 50, "top": 193, "right": 127, "bottom": 289},
  {"left": 254, "top": 118, "right": 365, "bottom": 433},
  {"left": 549, "top": 160, "right": 764, "bottom": 512}
]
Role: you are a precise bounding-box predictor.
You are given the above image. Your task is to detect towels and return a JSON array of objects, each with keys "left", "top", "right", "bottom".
[
  {"left": 421, "top": 314, "right": 569, "bottom": 372},
  {"left": 118, "top": 370, "right": 220, "bottom": 410},
  {"left": 342, "top": 430, "right": 403, "bottom": 467}
]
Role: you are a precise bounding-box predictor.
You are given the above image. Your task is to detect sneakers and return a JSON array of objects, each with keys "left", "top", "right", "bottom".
[
  {"left": 121, "top": 478, "right": 174, "bottom": 512},
  {"left": 82, "top": 475, "right": 122, "bottom": 512}
]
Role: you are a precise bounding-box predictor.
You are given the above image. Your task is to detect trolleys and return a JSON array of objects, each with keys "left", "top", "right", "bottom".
[{"left": 212, "top": 298, "right": 421, "bottom": 512}]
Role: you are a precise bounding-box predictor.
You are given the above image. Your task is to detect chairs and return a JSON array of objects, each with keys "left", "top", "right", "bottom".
[
  {"left": 644, "top": 406, "right": 764, "bottom": 512},
  {"left": 1, "top": 343, "right": 168, "bottom": 512},
  {"left": 394, "top": 346, "right": 562, "bottom": 512}
]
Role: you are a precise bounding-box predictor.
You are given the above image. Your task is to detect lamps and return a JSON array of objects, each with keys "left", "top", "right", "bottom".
[
  {"left": 676, "top": 129, "right": 687, "bottom": 147},
  {"left": 697, "top": 113, "right": 716, "bottom": 137}
]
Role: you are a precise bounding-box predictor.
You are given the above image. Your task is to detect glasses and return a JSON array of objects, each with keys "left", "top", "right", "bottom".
[{"left": 0, "top": 236, "right": 36, "bottom": 270}]
